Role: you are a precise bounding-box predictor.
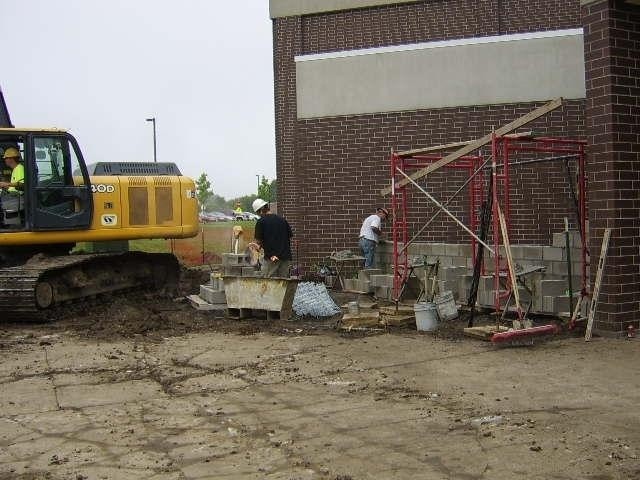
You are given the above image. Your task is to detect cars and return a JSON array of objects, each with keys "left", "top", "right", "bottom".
[{"left": 202, "top": 211, "right": 261, "bottom": 221}]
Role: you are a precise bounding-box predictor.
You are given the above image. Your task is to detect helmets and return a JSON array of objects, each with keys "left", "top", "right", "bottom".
[
  {"left": 3, "top": 147, "right": 21, "bottom": 158},
  {"left": 253, "top": 198, "right": 268, "bottom": 211},
  {"left": 377, "top": 207, "right": 389, "bottom": 215}
]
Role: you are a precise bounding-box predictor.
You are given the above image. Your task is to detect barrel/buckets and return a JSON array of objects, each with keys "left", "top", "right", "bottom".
[
  {"left": 413, "top": 302, "right": 439, "bottom": 332},
  {"left": 433, "top": 290, "right": 459, "bottom": 322},
  {"left": 348, "top": 301, "right": 359, "bottom": 315}
]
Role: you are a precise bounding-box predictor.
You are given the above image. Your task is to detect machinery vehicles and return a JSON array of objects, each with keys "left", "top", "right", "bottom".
[{"left": 0, "top": 88, "right": 198, "bottom": 324}]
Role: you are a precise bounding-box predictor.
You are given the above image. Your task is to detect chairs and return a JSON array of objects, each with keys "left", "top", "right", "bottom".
[{"left": 0, "top": 161, "right": 38, "bottom": 213}]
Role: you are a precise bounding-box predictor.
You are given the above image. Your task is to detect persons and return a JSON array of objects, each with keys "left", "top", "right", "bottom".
[
  {"left": 359, "top": 207, "right": 389, "bottom": 269},
  {"left": 0, "top": 147, "right": 25, "bottom": 211},
  {"left": 235, "top": 204, "right": 242, "bottom": 212},
  {"left": 252, "top": 198, "right": 293, "bottom": 277}
]
